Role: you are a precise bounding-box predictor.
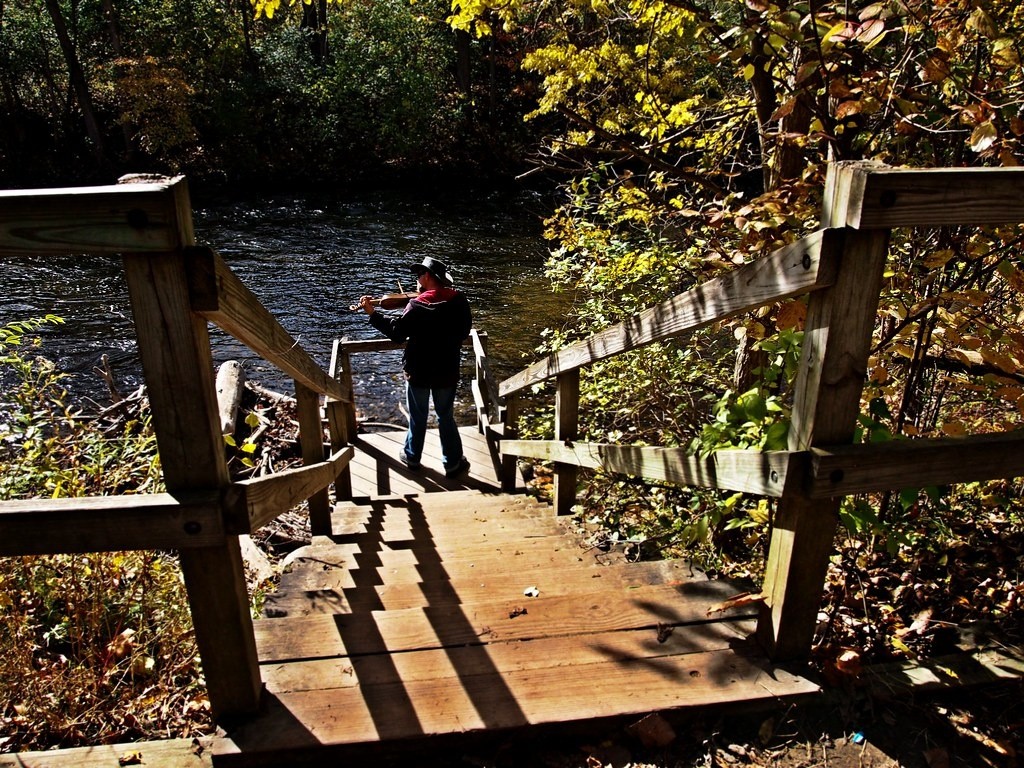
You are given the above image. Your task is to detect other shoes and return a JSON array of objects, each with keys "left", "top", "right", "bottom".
[
  {"left": 444, "top": 462, "right": 470, "bottom": 479},
  {"left": 400, "top": 449, "right": 420, "bottom": 468}
]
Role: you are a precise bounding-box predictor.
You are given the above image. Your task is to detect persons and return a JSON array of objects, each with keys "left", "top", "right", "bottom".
[{"left": 360, "top": 256, "right": 473, "bottom": 475}]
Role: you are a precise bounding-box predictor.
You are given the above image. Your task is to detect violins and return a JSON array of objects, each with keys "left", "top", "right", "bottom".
[{"left": 348, "top": 291, "right": 423, "bottom": 311}]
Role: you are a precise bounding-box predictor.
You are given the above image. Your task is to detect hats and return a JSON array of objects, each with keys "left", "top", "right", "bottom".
[{"left": 410, "top": 256, "right": 453, "bottom": 286}]
